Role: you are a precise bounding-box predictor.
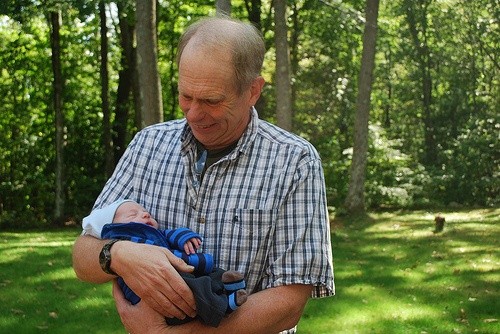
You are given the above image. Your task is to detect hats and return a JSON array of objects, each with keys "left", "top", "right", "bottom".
[{"left": 81, "top": 199, "right": 136, "bottom": 240}]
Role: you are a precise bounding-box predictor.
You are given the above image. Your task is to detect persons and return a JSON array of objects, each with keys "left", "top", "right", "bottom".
[
  {"left": 73, "top": 15, "right": 335, "bottom": 334},
  {"left": 82, "top": 199, "right": 248, "bottom": 329}
]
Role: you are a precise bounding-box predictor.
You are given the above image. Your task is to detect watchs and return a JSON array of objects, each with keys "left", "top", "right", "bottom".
[{"left": 98, "top": 240, "right": 122, "bottom": 277}]
